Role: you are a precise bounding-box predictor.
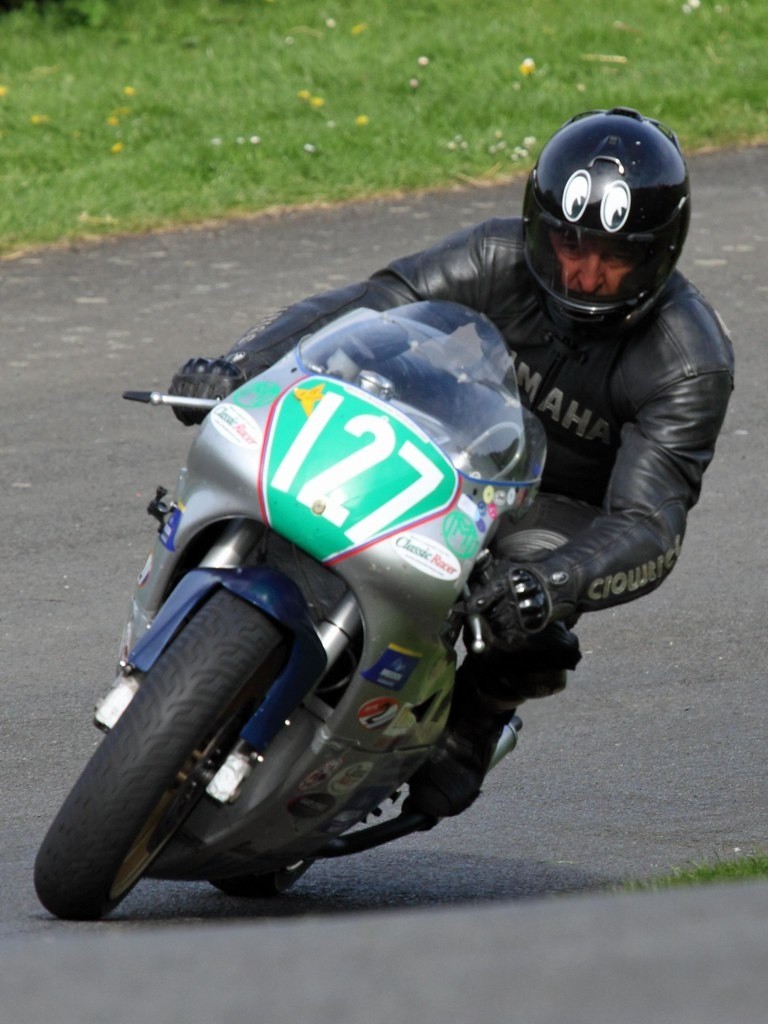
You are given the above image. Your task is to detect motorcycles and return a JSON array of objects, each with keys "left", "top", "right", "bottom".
[{"left": 34, "top": 301, "right": 523, "bottom": 921}]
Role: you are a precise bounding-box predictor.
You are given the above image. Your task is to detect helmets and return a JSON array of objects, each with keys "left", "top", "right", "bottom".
[{"left": 520, "top": 110, "right": 690, "bottom": 341}]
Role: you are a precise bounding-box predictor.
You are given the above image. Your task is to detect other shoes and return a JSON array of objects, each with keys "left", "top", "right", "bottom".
[{"left": 410, "top": 720, "right": 504, "bottom": 816}]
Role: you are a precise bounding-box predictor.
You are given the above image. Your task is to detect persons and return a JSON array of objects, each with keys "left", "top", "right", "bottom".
[{"left": 166, "top": 108, "right": 737, "bottom": 817}]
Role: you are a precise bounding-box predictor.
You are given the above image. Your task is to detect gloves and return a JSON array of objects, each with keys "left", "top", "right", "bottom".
[
  {"left": 467, "top": 560, "right": 550, "bottom": 650},
  {"left": 171, "top": 359, "right": 248, "bottom": 426}
]
